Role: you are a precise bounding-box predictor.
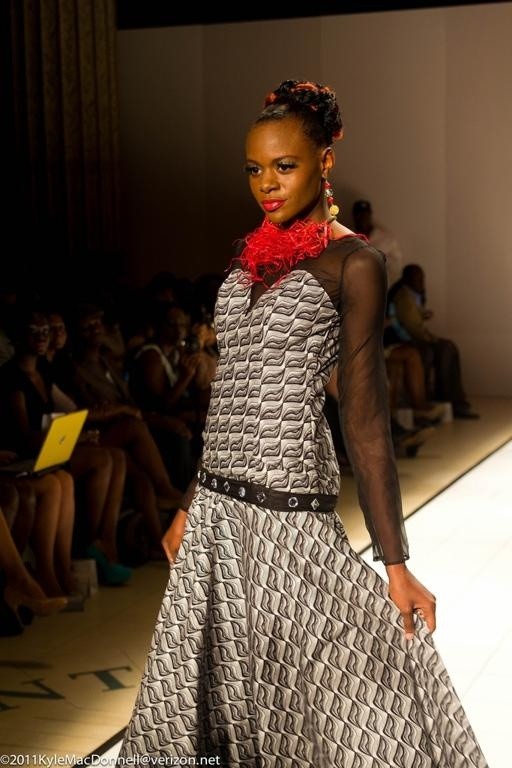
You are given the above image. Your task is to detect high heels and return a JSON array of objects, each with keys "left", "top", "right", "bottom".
[
  {"left": 415, "top": 405, "right": 447, "bottom": 421},
  {"left": 4, "top": 584, "right": 68, "bottom": 629},
  {"left": 86, "top": 544, "right": 135, "bottom": 584}
]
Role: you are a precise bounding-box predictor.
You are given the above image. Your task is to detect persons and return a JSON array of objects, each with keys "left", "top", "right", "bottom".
[
  {"left": 116, "top": 78, "right": 489, "bottom": 768},
  {"left": 0, "top": 198, "right": 481, "bottom": 636}
]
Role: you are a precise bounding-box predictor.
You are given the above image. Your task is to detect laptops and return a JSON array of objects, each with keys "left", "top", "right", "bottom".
[{"left": 0, "top": 408, "right": 89, "bottom": 479}]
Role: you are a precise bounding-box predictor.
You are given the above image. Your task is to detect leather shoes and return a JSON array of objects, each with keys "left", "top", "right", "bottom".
[{"left": 399, "top": 423, "right": 436, "bottom": 457}]
